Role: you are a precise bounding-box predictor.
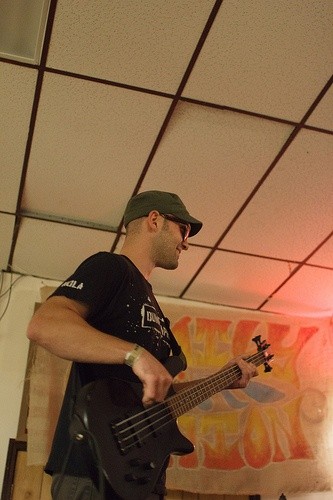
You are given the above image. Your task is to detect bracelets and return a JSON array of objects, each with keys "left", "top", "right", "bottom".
[{"left": 124, "top": 343, "right": 144, "bottom": 367}]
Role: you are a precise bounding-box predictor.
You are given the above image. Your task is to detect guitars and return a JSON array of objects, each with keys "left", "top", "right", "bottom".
[{"left": 67, "top": 334, "right": 274, "bottom": 500}]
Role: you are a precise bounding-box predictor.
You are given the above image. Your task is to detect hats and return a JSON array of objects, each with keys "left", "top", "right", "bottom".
[{"left": 124, "top": 189, "right": 203, "bottom": 236}]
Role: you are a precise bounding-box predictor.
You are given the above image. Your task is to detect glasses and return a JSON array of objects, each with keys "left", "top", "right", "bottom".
[{"left": 160, "top": 214, "right": 192, "bottom": 242}]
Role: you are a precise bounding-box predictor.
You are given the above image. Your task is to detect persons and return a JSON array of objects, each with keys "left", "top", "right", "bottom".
[{"left": 26, "top": 191, "right": 259, "bottom": 500}]
